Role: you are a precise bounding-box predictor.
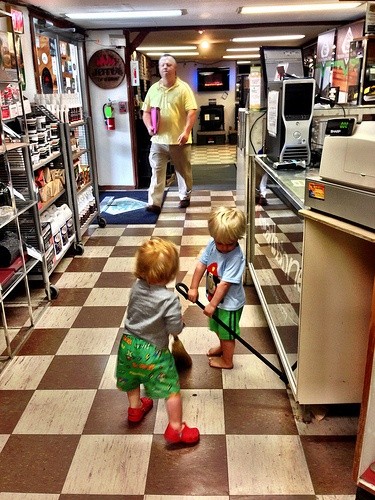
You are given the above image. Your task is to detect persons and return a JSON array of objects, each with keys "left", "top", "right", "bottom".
[
  {"left": 142, "top": 54, "right": 197, "bottom": 213},
  {"left": 119, "top": 238, "right": 201, "bottom": 444},
  {"left": 188, "top": 205, "right": 246, "bottom": 368}
]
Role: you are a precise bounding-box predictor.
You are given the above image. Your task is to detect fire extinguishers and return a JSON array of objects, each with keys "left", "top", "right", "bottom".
[{"left": 103, "top": 98, "right": 116, "bottom": 131}]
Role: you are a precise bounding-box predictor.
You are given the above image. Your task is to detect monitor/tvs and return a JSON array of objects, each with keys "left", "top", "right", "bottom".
[{"left": 197, "top": 68, "right": 229, "bottom": 91}]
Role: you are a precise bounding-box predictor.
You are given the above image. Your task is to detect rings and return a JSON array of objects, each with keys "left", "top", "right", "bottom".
[{"left": 186, "top": 141, "right": 187, "bottom": 142}]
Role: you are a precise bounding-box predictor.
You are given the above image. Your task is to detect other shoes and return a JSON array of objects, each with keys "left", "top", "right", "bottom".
[
  {"left": 146, "top": 206, "right": 162, "bottom": 214},
  {"left": 180, "top": 199, "right": 191, "bottom": 208},
  {"left": 127, "top": 396, "right": 152, "bottom": 422},
  {"left": 164, "top": 423, "right": 200, "bottom": 444}
]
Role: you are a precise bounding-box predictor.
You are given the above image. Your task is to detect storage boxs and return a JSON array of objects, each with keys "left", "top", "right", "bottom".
[{"left": 0, "top": 94, "right": 31, "bottom": 118}]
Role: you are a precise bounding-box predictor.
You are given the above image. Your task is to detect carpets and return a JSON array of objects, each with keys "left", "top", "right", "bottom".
[{"left": 91, "top": 191, "right": 168, "bottom": 225}]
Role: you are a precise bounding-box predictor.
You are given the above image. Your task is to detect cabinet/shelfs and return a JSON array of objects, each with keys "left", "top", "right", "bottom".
[
  {"left": 236, "top": 108, "right": 375, "bottom": 424},
  {"left": 0, "top": 103, "right": 107, "bottom": 376}
]
